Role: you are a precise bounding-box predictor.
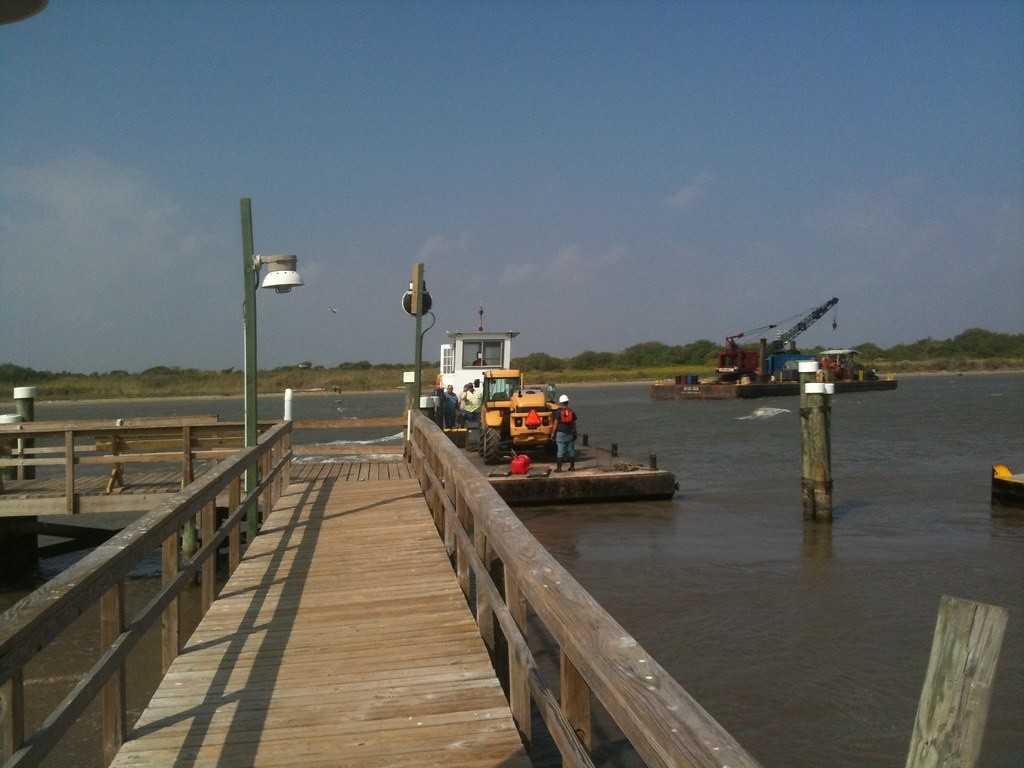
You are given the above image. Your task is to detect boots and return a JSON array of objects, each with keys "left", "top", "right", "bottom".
[
  {"left": 568, "top": 458, "right": 575, "bottom": 471},
  {"left": 554, "top": 459, "right": 563, "bottom": 472}
]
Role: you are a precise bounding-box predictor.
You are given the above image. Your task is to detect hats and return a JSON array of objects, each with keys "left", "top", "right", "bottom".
[{"left": 467, "top": 383, "right": 473, "bottom": 388}]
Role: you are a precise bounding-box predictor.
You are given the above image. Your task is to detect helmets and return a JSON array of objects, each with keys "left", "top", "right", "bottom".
[{"left": 559, "top": 395, "right": 569, "bottom": 403}]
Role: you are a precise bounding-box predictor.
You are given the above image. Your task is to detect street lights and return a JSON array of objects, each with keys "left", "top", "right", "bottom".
[{"left": 238, "top": 196, "right": 304, "bottom": 541}]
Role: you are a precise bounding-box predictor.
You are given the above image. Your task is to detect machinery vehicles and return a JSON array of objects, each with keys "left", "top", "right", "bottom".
[{"left": 463, "top": 368, "right": 574, "bottom": 466}]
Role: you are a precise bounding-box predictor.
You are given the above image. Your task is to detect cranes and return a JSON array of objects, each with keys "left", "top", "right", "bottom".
[{"left": 715, "top": 297, "right": 840, "bottom": 381}]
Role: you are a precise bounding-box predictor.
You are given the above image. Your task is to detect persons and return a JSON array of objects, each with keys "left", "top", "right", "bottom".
[
  {"left": 472, "top": 351, "right": 487, "bottom": 366},
  {"left": 442, "top": 382, "right": 483, "bottom": 428},
  {"left": 550, "top": 395, "right": 578, "bottom": 472}
]
